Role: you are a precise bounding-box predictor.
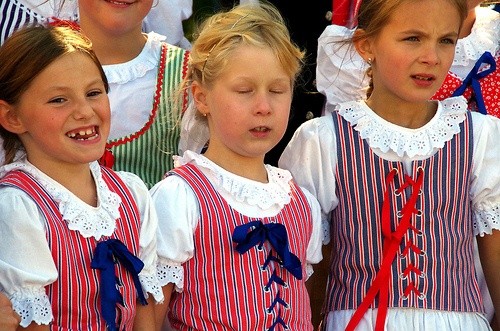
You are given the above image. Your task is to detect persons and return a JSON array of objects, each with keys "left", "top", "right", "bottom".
[
  {"left": 0, "top": 0, "right": 500, "bottom": 331},
  {"left": 143, "top": 0, "right": 323, "bottom": 331}
]
